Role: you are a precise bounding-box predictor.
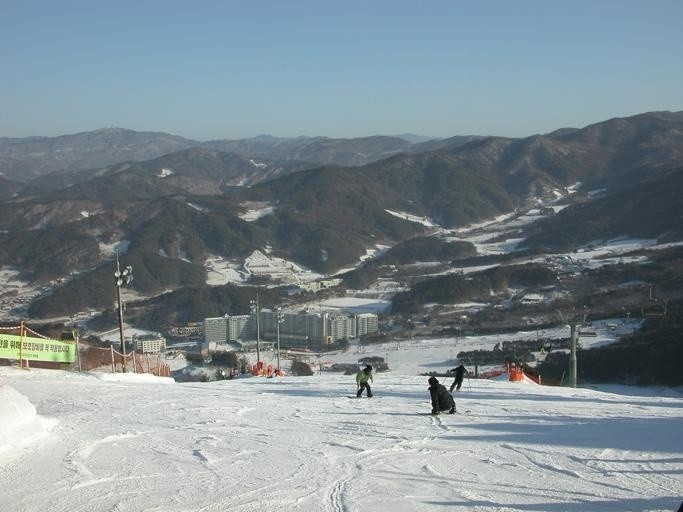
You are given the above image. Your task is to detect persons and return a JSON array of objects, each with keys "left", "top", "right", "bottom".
[
  {"left": 427, "top": 376, "right": 457, "bottom": 414},
  {"left": 354, "top": 364, "right": 375, "bottom": 399},
  {"left": 447, "top": 360, "right": 470, "bottom": 391}
]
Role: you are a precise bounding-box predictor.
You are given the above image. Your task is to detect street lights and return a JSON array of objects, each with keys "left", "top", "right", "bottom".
[
  {"left": 115, "top": 265, "right": 134, "bottom": 374},
  {"left": 248, "top": 297, "right": 260, "bottom": 364},
  {"left": 274, "top": 312, "right": 285, "bottom": 370}
]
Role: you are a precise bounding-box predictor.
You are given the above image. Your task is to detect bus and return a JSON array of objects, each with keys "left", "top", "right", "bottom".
[{"left": 579, "top": 332, "right": 596, "bottom": 337}]
